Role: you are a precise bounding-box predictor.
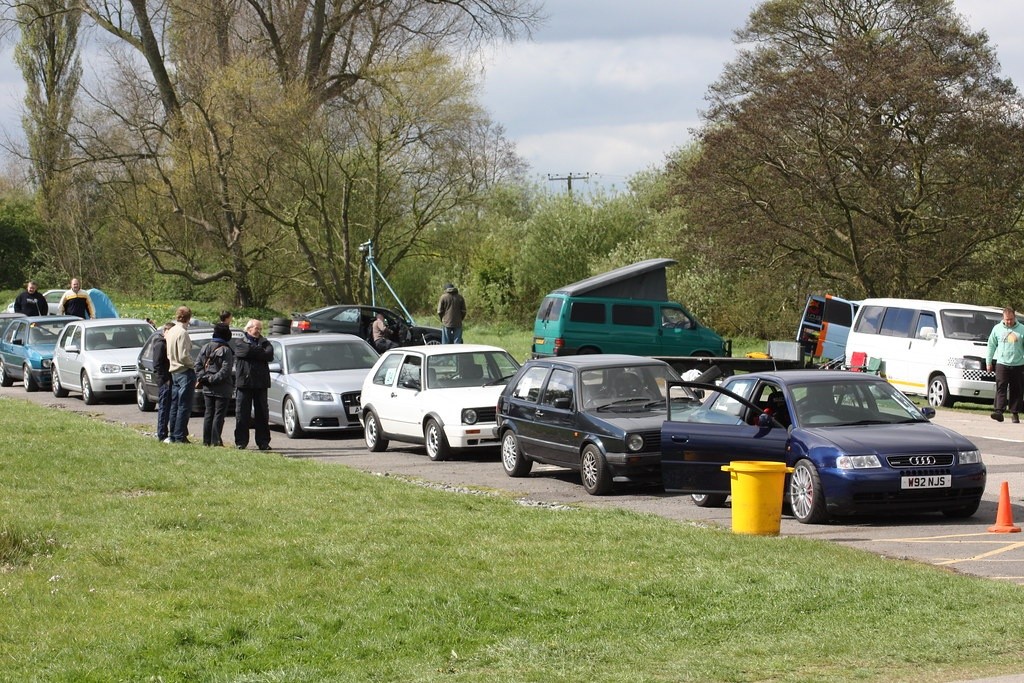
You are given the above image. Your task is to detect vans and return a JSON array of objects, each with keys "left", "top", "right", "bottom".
[
  {"left": 531, "top": 258, "right": 733, "bottom": 361},
  {"left": 796, "top": 293, "right": 1024, "bottom": 408}
]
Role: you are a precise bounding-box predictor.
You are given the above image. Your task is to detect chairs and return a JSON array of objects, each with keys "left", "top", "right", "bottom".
[
  {"left": 291, "top": 349, "right": 306, "bottom": 363},
  {"left": 612, "top": 373, "right": 641, "bottom": 394},
  {"left": 419, "top": 368, "right": 436, "bottom": 383},
  {"left": 463, "top": 364, "right": 483, "bottom": 380},
  {"left": 766, "top": 391, "right": 790, "bottom": 422},
  {"left": 844, "top": 352, "right": 884, "bottom": 404},
  {"left": 112, "top": 331, "right": 138, "bottom": 343},
  {"left": 88, "top": 332, "right": 107, "bottom": 345}
]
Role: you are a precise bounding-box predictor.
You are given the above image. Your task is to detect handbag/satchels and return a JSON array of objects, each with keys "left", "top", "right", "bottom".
[{"left": 194, "top": 370, "right": 207, "bottom": 389}]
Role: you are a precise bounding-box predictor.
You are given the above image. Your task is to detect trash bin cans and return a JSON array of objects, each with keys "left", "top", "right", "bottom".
[{"left": 721, "top": 460, "right": 795, "bottom": 535}]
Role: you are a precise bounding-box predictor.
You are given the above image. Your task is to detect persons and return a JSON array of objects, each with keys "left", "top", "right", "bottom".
[
  {"left": 153, "top": 323, "right": 176, "bottom": 443},
  {"left": 14, "top": 282, "right": 49, "bottom": 318},
  {"left": 373, "top": 314, "right": 399, "bottom": 352},
  {"left": 437, "top": 283, "right": 466, "bottom": 345},
  {"left": 218, "top": 311, "right": 232, "bottom": 325},
  {"left": 166, "top": 307, "right": 197, "bottom": 443},
  {"left": 987, "top": 307, "right": 1024, "bottom": 424},
  {"left": 234, "top": 320, "right": 274, "bottom": 449},
  {"left": 194, "top": 324, "right": 234, "bottom": 447},
  {"left": 56, "top": 278, "right": 96, "bottom": 321}
]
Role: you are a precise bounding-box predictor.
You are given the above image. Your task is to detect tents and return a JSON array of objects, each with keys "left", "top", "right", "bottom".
[{"left": 83, "top": 289, "right": 120, "bottom": 318}]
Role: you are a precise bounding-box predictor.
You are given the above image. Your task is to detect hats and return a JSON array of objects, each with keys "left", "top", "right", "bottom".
[
  {"left": 213, "top": 324, "right": 232, "bottom": 342},
  {"left": 443, "top": 284, "right": 454, "bottom": 290}
]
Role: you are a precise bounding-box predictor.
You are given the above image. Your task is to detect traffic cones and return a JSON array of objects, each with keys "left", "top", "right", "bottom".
[{"left": 987, "top": 481, "right": 1022, "bottom": 534}]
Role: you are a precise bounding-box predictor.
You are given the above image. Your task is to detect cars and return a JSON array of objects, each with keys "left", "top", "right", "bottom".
[
  {"left": 659, "top": 368, "right": 987, "bottom": 524},
  {"left": 251, "top": 333, "right": 382, "bottom": 439},
  {"left": 289, "top": 305, "right": 464, "bottom": 356},
  {"left": 0, "top": 289, "right": 87, "bottom": 393},
  {"left": 492, "top": 354, "right": 704, "bottom": 495},
  {"left": 133, "top": 327, "right": 251, "bottom": 416},
  {"left": 356, "top": 343, "right": 522, "bottom": 462},
  {"left": 50, "top": 317, "right": 157, "bottom": 405}
]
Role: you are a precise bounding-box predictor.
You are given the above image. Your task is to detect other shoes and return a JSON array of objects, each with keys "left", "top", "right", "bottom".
[
  {"left": 175, "top": 437, "right": 192, "bottom": 444},
  {"left": 237, "top": 443, "right": 245, "bottom": 449},
  {"left": 159, "top": 435, "right": 168, "bottom": 442},
  {"left": 991, "top": 413, "right": 1004, "bottom": 422},
  {"left": 259, "top": 443, "right": 272, "bottom": 450},
  {"left": 1012, "top": 414, "right": 1019, "bottom": 423}
]
